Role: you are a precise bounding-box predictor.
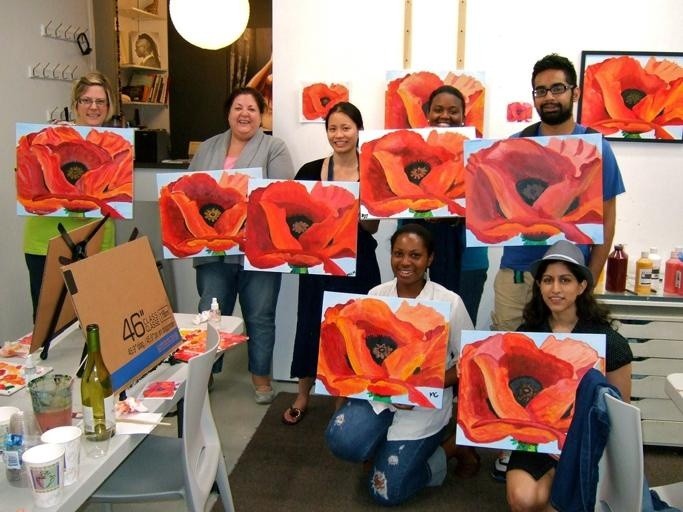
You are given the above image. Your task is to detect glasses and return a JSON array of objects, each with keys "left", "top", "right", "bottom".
[{"left": 532, "top": 84, "right": 574, "bottom": 98}]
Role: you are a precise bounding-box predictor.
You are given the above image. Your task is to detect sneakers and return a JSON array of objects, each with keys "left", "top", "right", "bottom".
[
  {"left": 491, "top": 456, "right": 510, "bottom": 480},
  {"left": 255, "top": 384, "right": 274, "bottom": 403}
]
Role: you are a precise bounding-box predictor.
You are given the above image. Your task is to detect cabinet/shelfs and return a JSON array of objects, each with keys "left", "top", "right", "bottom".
[
  {"left": 580, "top": 289, "right": 683, "bottom": 451},
  {"left": 92, "top": 1, "right": 174, "bottom": 165}
]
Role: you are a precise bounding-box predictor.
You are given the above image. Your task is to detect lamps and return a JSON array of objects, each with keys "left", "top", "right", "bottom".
[{"left": 168, "top": 0, "right": 252, "bottom": 51}]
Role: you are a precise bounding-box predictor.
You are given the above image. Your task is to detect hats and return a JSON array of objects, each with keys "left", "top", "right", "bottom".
[{"left": 531, "top": 240, "right": 593, "bottom": 289}]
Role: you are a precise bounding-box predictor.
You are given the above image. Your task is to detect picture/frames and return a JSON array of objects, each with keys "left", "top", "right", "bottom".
[{"left": 573, "top": 51, "right": 683, "bottom": 145}]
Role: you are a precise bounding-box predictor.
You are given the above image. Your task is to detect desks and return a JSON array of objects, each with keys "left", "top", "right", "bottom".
[
  {"left": 662, "top": 368, "right": 683, "bottom": 409},
  {"left": 0, "top": 305, "right": 251, "bottom": 512}
]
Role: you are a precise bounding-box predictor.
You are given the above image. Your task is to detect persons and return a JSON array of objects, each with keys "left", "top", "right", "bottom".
[
  {"left": 398, "top": 86, "right": 489, "bottom": 326},
  {"left": 245, "top": 55, "right": 272, "bottom": 130},
  {"left": 326, "top": 225, "right": 479, "bottom": 507},
  {"left": 135, "top": 33, "right": 160, "bottom": 68},
  {"left": 491, "top": 55, "right": 624, "bottom": 478},
  {"left": 187, "top": 89, "right": 295, "bottom": 405},
  {"left": 282, "top": 101, "right": 383, "bottom": 422},
  {"left": 500, "top": 241, "right": 634, "bottom": 511},
  {"left": 24, "top": 74, "right": 115, "bottom": 328}
]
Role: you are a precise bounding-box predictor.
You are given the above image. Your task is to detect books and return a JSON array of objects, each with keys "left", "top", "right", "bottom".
[{"left": 124, "top": 73, "right": 170, "bottom": 103}]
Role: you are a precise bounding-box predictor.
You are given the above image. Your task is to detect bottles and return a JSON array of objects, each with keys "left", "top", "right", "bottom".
[
  {"left": 635, "top": 252, "right": 651, "bottom": 293},
  {"left": 80, "top": 324, "right": 117, "bottom": 441},
  {"left": 648, "top": 247, "right": 661, "bottom": 292},
  {"left": 603, "top": 244, "right": 627, "bottom": 293},
  {"left": 663, "top": 247, "right": 682, "bottom": 298},
  {"left": 208, "top": 296, "right": 221, "bottom": 330}
]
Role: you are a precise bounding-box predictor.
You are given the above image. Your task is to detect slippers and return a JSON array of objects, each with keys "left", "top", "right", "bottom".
[{"left": 282, "top": 405, "right": 304, "bottom": 425}]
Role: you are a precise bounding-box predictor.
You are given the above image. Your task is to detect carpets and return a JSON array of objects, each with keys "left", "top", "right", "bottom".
[{"left": 204, "top": 381, "right": 683, "bottom": 512}]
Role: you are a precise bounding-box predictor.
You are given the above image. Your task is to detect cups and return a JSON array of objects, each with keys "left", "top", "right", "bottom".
[
  {"left": 22, "top": 442, "right": 66, "bottom": 509},
  {"left": 27, "top": 373, "right": 74, "bottom": 432},
  {"left": 41, "top": 424, "right": 80, "bottom": 485},
  {"left": 76, "top": 418, "right": 114, "bottom": 459},
  {"left": 0, "top": 413, "right": 27, "bottom": 485}
]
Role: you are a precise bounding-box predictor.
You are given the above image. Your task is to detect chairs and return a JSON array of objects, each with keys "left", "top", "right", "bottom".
[
  {"left": 592, "top": 386, "right": 682, "bottom": 512},
  {"left": 81, "top": 319, "right": 238, "bottom": 512}
]
